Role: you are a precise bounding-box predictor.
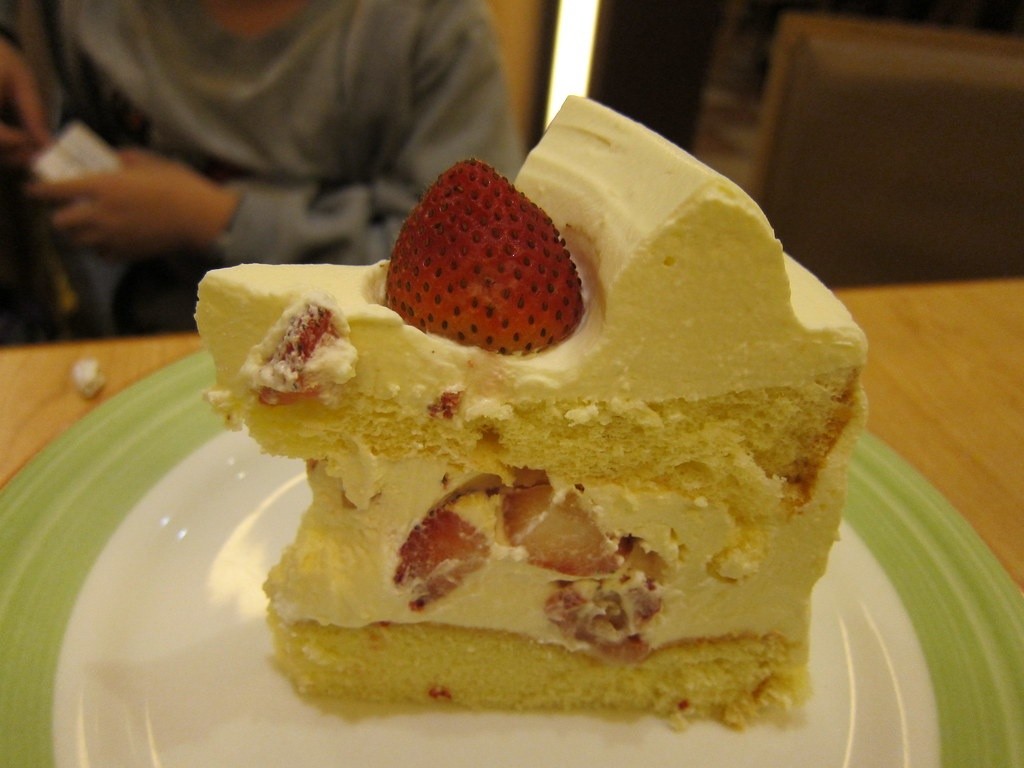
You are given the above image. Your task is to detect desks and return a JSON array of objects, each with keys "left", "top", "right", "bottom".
[{"left": 1, "top": 278, "right": 1022, "bottom": 593}]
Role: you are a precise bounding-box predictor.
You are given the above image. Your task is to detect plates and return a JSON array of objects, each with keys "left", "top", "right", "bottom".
[{"left": 0, "top": 347, "right": 1023, "bottom": 768}]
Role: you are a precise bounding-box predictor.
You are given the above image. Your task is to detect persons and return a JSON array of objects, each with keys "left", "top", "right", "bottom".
[{"left": 0, "top": 1, "right": 508, "bottom": 340}]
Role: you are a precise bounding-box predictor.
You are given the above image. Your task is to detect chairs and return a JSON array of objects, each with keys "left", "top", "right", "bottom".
[{"left": 744, "top": 13, "right": 1024, "bottom": 288}]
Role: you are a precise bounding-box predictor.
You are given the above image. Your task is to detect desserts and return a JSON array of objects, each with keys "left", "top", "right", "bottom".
[{"left": 192, "top": 97, "right": 871, "bottom": 734}]
